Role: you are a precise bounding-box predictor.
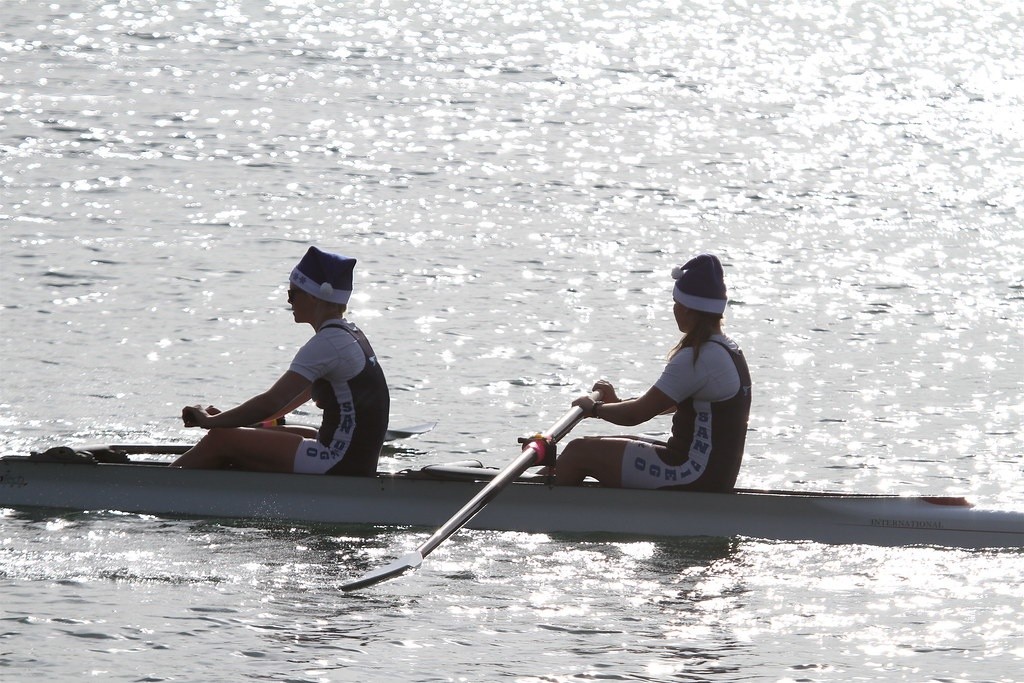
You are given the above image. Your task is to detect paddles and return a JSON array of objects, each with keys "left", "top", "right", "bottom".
[
  {"left": 258, "top": 422, "right": 440, "bottom": 442},
  {"left": 334, "top": 390, "right": 606, "bottom": 592}
]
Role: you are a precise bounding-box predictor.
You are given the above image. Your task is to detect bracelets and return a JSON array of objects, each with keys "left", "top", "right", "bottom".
[{"left": 592, "top": 399, "right": 604, "bottom": 418}]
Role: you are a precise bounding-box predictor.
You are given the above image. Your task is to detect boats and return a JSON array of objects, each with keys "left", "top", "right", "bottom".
[{"left": 2, "top": 440, "right": 1024, "bottom": 556}]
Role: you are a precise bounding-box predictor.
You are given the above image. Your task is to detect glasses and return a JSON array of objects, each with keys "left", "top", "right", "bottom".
[{"left": 288, "top": 288, "right": 306, "bottom": 304}]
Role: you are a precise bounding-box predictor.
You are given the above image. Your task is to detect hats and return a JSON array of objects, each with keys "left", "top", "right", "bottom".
[
  {"left": 289, "top": 246, "right": 357, "bottom": 304},
  {"left": 671, "top": 253, "right": 728, "bottom": 314}
]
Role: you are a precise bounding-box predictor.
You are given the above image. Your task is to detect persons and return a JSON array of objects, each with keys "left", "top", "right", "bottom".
[
  {"left": 168, "top": 246, "right": 391, "bottom": 475},
  {"left": 534, "top": 254, "right": 752, "bottom": 492}
]
